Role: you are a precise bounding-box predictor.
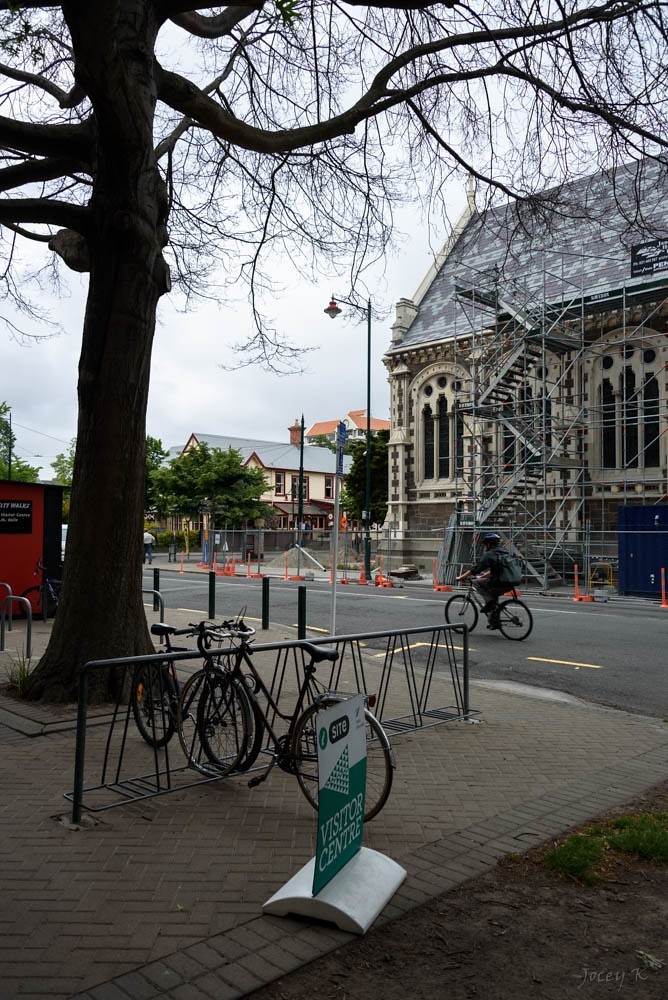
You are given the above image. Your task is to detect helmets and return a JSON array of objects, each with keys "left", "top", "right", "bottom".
[{"left": 482, "top": 533, "right": 501, "bottom": 540}]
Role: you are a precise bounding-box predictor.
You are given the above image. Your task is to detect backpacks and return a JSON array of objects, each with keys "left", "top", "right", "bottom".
[{"left": 496, "top": 551, "right": 523, "bottom": 585}]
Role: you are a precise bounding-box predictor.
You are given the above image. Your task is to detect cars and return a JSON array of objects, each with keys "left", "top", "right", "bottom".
[{"left": 60, "top": 524, "right": 67, "bottom": 562}]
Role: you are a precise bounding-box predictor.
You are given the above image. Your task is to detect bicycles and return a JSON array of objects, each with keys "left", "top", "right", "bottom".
[
  {"left": 445, "top": 576, "right": 534, "bottom": 643},
  {"left": 177, "top": 625, "right": 397, "bottom": 826},
  {"left": 19, "top": 559, "right": 61, "bottom": 621},
  {"left": 128, "top": 621, "right": 266, "bottom": 780}
]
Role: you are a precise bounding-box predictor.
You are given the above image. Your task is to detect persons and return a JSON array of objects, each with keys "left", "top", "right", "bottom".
[
  {"left": 143, "top": 528, "right": 155, "bottom": 564},
  {"left": 455, "top": 533, "right": 509, "bottom": 628}
]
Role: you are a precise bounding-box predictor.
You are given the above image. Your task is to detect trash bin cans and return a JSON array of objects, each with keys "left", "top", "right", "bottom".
[{"left": 168, "top": 544, "right": 178, "bottom": 554}]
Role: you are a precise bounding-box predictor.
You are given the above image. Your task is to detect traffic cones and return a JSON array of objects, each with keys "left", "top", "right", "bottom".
[{"left": 224, "top": 560, "right": 230, "bottom": 572}]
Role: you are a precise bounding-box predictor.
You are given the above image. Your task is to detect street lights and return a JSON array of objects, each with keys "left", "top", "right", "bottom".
[{"left": 323, "top": 295, "right": 373, "bottom": 582}]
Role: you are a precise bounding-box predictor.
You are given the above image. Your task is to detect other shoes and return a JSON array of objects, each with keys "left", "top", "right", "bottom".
[
  {"left": 486, "top": 624, "right": 491, "bottom": 629},
  {"left": 480, "top": 602, "right": 496, "bottom": 613}
]
